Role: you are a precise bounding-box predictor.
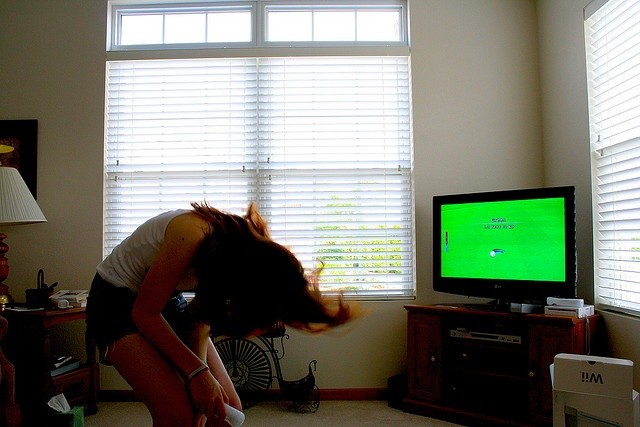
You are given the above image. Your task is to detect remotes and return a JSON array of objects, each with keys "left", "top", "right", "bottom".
[{"left": 225, "top": 404, "right": 244, "bottom": 427}]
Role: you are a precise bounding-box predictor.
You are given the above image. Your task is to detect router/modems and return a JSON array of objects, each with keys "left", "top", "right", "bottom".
[{"left": 545, "top": 306, "right": 597, "bottom": 317}]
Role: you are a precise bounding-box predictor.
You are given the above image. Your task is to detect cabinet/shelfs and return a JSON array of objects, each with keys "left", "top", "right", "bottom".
[
  {"left": 401, "top": 303, "right": 600, "bottom": 426},
  {"left": 3, "top": 306, "right": 99, "bottom": 426}
]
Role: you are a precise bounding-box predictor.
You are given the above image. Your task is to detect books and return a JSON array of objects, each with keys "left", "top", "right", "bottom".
[
  {"left": 50, "top": 287, "right": 88, "bottom": 308},
  {"left": 48, "top": 352, "right": 82, "bottom": 380}
]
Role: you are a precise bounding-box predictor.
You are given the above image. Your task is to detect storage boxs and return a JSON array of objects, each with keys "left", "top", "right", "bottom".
[
  {"left": 549, "top": 353, "right": 640, "bottom": 427},
  {"left": 52, "top": 405, "right": 84, "bottom": 427}
]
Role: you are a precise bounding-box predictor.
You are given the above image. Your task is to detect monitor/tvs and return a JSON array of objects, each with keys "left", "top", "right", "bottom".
[{"left": 432, "top": 186, "right": 576, "bottom": 312}]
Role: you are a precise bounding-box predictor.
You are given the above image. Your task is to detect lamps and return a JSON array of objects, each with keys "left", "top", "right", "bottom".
[{"left": 0, "top": 166, "right": 48, "bottom": 308}]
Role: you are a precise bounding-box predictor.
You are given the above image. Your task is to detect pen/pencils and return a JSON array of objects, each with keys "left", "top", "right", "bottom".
[{"left": 46, "top": 282, "right": 58, "bottom": 291}]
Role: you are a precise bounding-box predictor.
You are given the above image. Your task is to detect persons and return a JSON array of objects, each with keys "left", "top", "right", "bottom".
[{"left": 85, "top": 202, "right": 379, "bottom": 427}]
